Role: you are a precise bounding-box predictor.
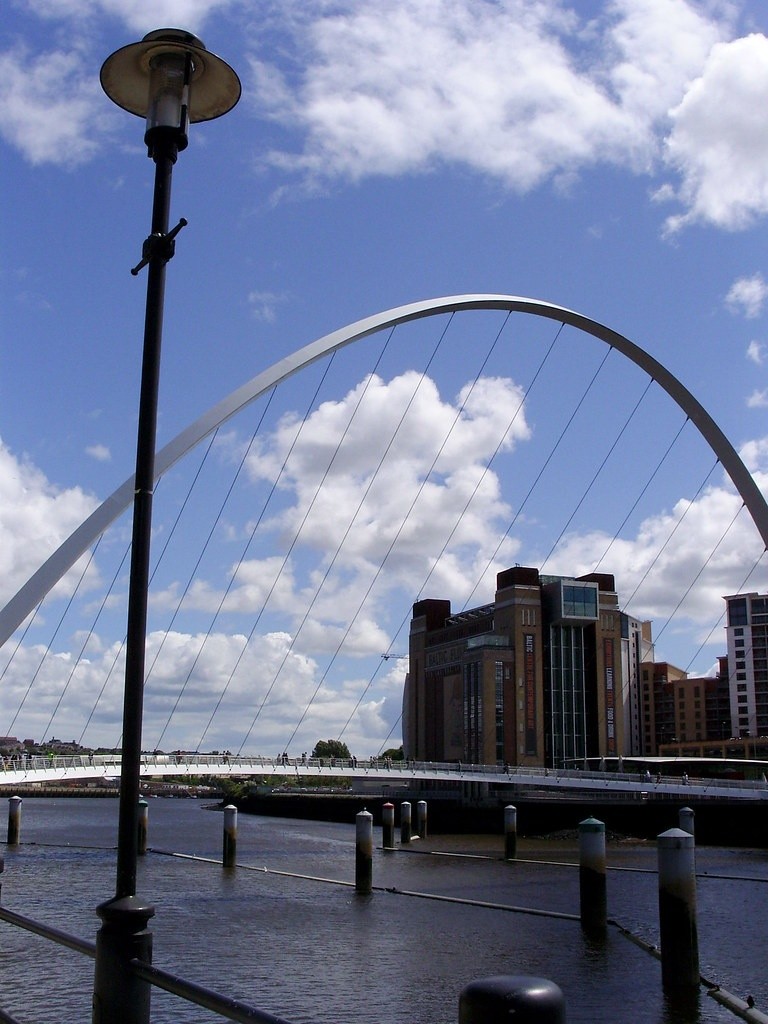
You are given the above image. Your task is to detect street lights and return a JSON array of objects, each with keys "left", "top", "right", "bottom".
[{"left": 89, "top": 26, "right": 244, "bottom": 1024}]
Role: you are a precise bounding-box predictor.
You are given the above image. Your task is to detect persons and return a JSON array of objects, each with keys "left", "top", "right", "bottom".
[
  {"left": 411, "top": 757, "right": 416, "bottom": 769},
  {"left": 350, "top": 756, "right": 357, "bottom": 767},
  {"left": 176, "top": 750, "right": 182, "bottom": 764},
  {"left": 457, "top": 760, "right": 461, "bottom": 771},
  {"left": 48, "top": 752, "right": 54, "bottom": 768},
  {"left": 299, "top": 752, "right": 308, "bottom": 766},
  {"left": 277, "top": 754, "right": 281, "bottom": 765},
  {"left": 87, "top": 749, "right": 94, "bottom": 765},
  {"left": 682, "top": 772, "right": 688, "bottom": 785},
  {"left": 282, "top": 752, "right": 290, "bottom": 766},
  {"left": 406, "top": 755, "right": 409, "bottom": 769},
  {"left": 502, "top": 760, "right": 508, "bottom": 774},
  {"left": 0, "top": 752, "right": 33, "bottom": 771},
  {"left": 383, "top": 757, "right": 392, "bottom": 769},
  {"left": 331, "top": 755, "right": 336, "bottom": 767},
  {"left": 153, "top": 749, "right": 158, "bottom": 756},
  {"left": 220, "top": 750, "right": 231, "bottom": 764},
  {"left": 370, "top": 756, "right": 375, "bottom": 768}
]
